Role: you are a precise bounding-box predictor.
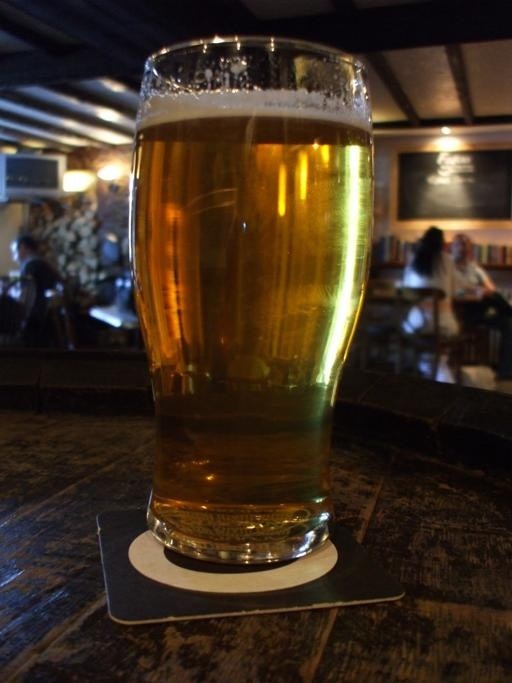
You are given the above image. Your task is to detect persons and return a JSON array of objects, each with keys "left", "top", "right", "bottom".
[
  {"left": 447, "top": 232, "right": 510, "bottom": 381},
  {"left": 401, "top": 227, "right": 460, "bottom": 384},
  {"left": 9, "top": 232, "right": 61, "bottom": 341}
]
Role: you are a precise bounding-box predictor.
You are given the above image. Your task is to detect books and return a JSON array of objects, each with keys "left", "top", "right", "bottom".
[{"left": 379, "top": 234, "right": 511, "bottom": 265}]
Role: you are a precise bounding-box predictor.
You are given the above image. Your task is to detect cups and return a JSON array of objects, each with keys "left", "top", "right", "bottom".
[{"left": 127, "top": 37, "right": 377, "bottom": 567}]
